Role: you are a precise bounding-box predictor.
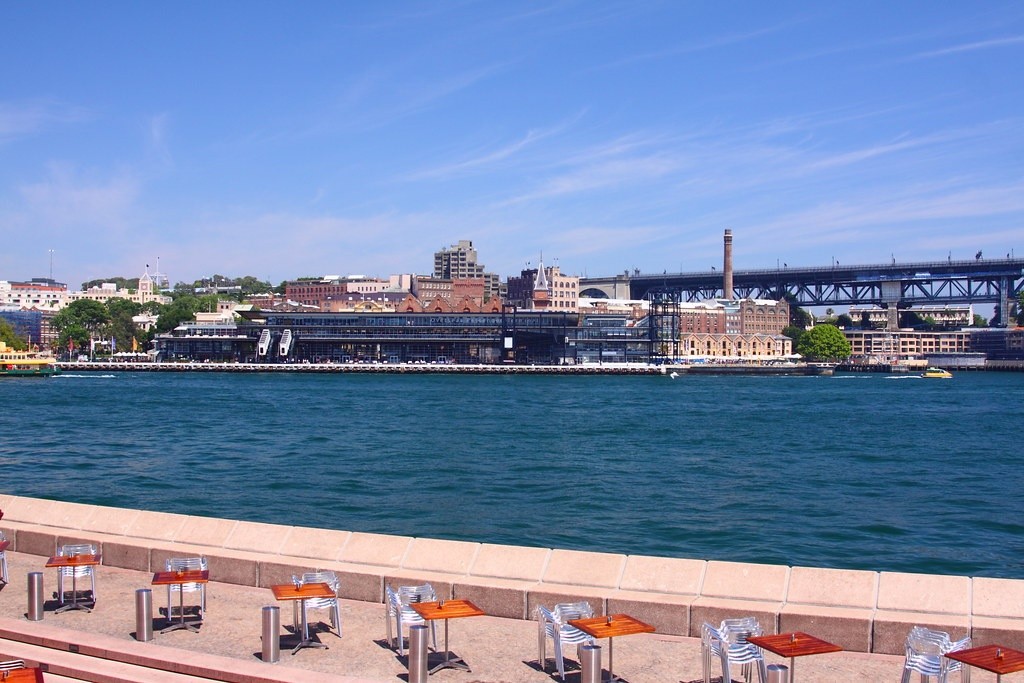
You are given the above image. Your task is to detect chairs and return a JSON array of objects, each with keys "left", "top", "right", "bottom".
[{"left": 0, "top": 542, "right": 972, "bottom": 683}]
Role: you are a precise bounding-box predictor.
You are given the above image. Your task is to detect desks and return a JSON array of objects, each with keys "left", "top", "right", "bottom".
[
  {"left": 45, "top": 555, "right": 102, "bottom": 614},
  {"left": 567, "top": 614, "right": 655, "bottom": 683},
  {"left": 409, "top": 599, "right": 486, "bottom": 675},
  {"left": 151, "top": 570, "right": 209, "bottom": 633},
  {"left": 944, "top": 644, "right": 1024, "bottom": 683},
  {"left": 0, "top": 666, "right": 45, "bottom": 683},
  {"left": 745, "top": 632, "right": 843, "bottom": 683},
  {"left": 271, "top": 583, "right": 336, "bottom": 655}
]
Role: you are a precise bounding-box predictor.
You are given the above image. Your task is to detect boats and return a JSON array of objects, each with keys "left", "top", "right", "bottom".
[
  {"left": 921, "top": 367, "right": 954, "bottom": 378},
  {"left": 0, "top": 342, "right": 63, "bottom": 377}
]
